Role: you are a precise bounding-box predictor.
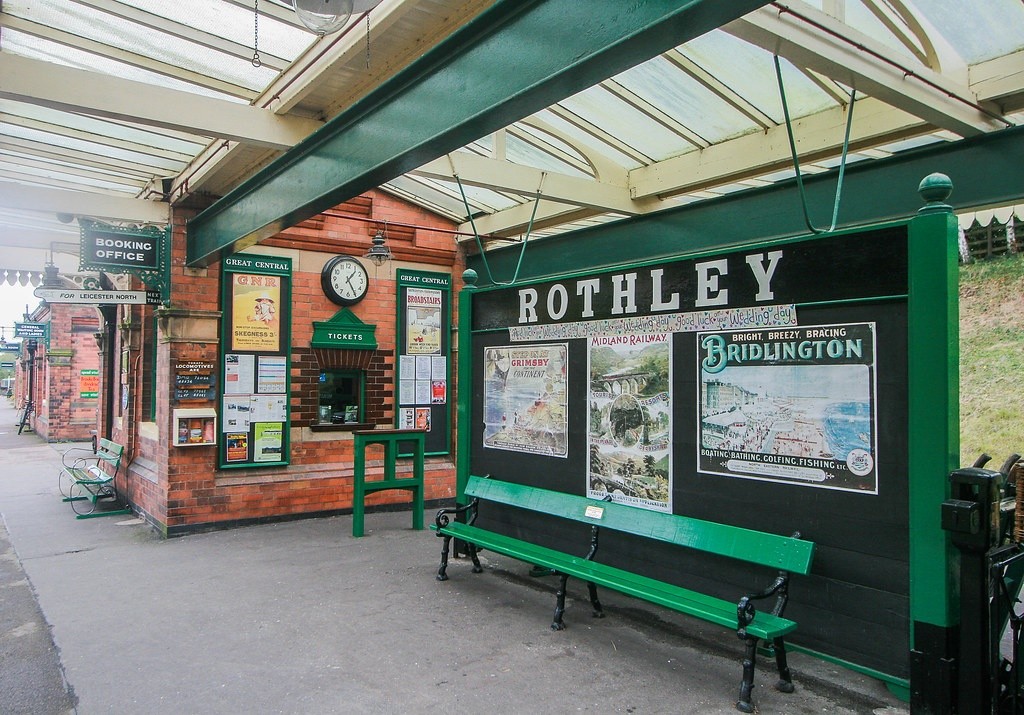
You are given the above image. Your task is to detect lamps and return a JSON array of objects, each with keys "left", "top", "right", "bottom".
[{"left": 364, "top": 223, "right": 394, "bottom": 266}]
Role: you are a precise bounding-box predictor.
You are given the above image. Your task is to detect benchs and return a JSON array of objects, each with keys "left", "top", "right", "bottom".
[
  {"left": 430, "top": 475, "right": 816, "bottom": 714},
  {"left": 58, "top": 438, "right": 133, "bottom": 519}
]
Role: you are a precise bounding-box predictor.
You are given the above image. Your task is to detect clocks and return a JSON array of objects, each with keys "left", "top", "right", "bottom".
[{"left": 321, "top": 256, "right": 369, "bottom": 307}]
